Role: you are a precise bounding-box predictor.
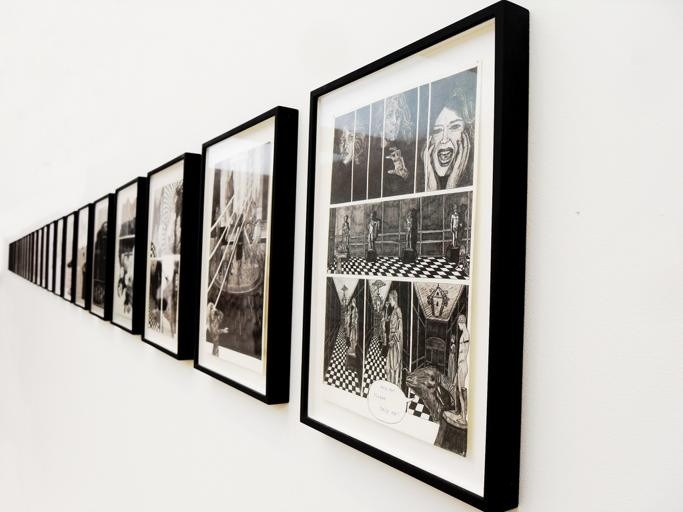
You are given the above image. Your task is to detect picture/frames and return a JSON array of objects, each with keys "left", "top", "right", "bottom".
[
  {"left": 32, "top": 152, "right": 201, "bottom": 360},
  {"left": 300, "top": 0, "right": 530, "bottom": 512},
  {"left": 194, "top": 105, "right": 300, "bottom": 405}
]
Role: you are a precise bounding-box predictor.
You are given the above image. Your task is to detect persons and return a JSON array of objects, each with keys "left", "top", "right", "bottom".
[
  {"left": 344, "top": 298, "right": 358, "bottom": 353},
  {"left": 421, "top": 87, "right": 471, "bottom": 192},
  {"left": 450, "top": 206, "right": 463, "bottom": 248},
  {"left": 380, "top": 291, "right": 402, "bottom": 388},
  {"left": 405, "top": 208, "right": 415, "bottom": 250},
  {"left": 337, "top": 117, "right": 369, "bottom": 198},
  {"left": 337, "top": 215, "right": 349, "bottom": 254},
  {"left": 369, "top": 96, "right": 413, "bottom": 195},
  {"left": 448, "top": 315, "right": 470, "bottom": 425},
  {"left": 366, "top": 211, "right": 379, "bottom": 253}
]
